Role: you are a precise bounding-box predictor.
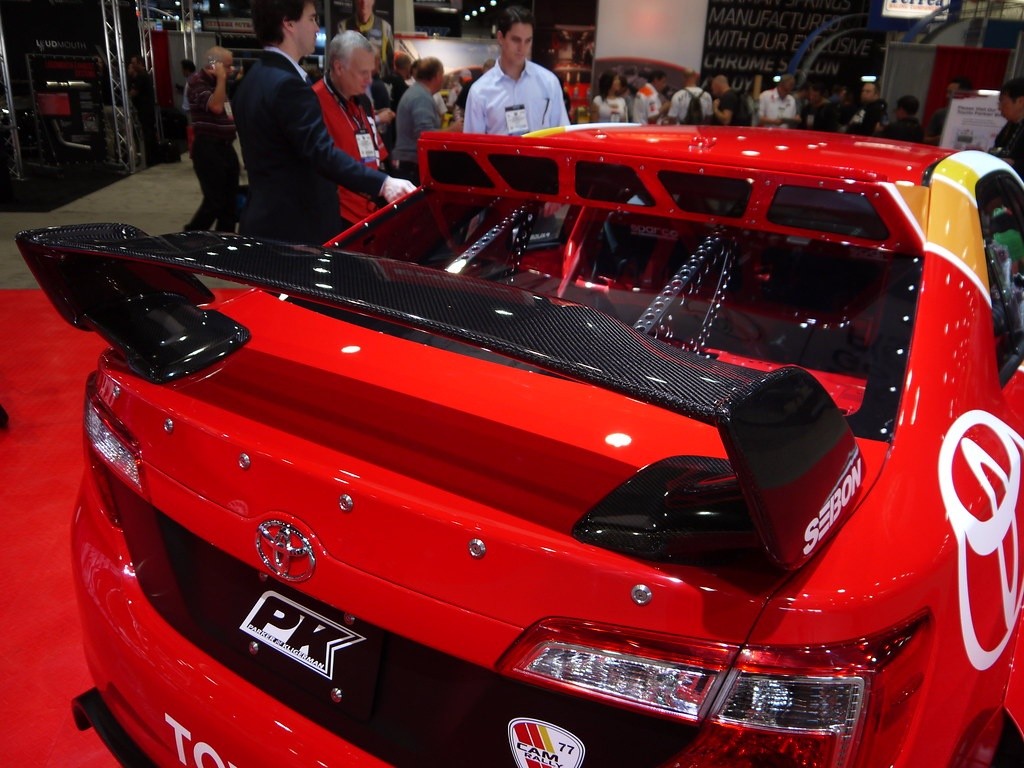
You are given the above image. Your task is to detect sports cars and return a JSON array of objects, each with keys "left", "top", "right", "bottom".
[{"left": 10, "top": 120, "right": 1024, "bottom": 768}]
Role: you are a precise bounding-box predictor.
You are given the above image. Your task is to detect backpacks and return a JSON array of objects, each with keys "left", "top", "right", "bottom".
[{"left": 677, "top": 87, "right": 706, "bottom": 126}]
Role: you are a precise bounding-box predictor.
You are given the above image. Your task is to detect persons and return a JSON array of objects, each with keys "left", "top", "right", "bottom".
[
  {"left": 364, "top": 53, "right": 449, "bottom": 186},
  {"left": 182, "top": 46, "right": 244, "bottom": 232},
  {"left": 334, "top": 0, "right": 394, "bottom": 86},
  {"left": 589, "top": 68, "right": 740, "bottom": 126},
  {"left": 231, "top": 0, "right": 417, "bottom": 250},
  {"left": 311, "top": 30, "right": 388, "bottom": 229},
  {"left": 463, "top": 7, "right": 570, "bottom": 136},
  {"left": 758, "top": 74, "right": 925, "bottom": 144},
  {"left": 128, "top": 55, "right": 159, "bottom": 165},
  {"left": 924, "top": 77, "right": 972, "bottom": 145},
  {"left": 453, "top": 58, "right": 496, "bottom": 120},
  {"left": 559, "top": 77, "right": 572, "bottom": 110},
  {"left": 966, "top": 78, "right": 1024, "bottom": 183}
]
